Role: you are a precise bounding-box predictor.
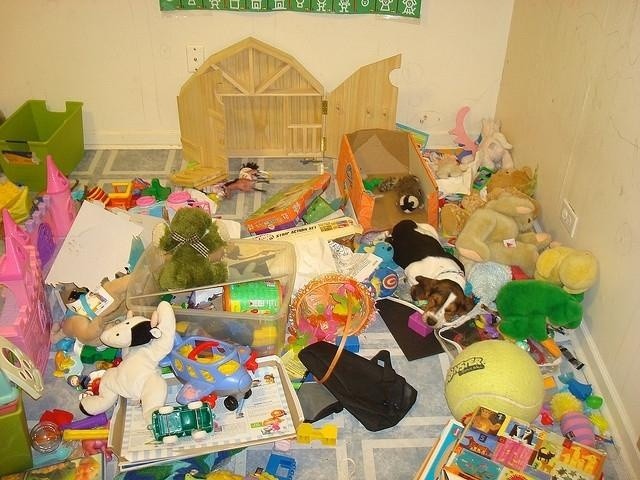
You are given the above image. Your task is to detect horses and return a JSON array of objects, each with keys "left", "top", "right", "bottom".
[{"left": 221, "top": 162, "right": 270, "bottom": 200}]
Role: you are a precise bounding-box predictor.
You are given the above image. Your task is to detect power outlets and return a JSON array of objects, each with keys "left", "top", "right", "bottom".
[{"left": 556, "top": 198, "right": 577, "bottom": 238}]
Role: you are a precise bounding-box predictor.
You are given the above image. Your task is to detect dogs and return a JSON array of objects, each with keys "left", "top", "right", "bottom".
[{"left": 388, "top": 219, "right": 476, "bottom": 328}]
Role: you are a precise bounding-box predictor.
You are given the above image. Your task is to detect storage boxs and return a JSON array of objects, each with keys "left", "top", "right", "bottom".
[
  {"left": 125, "top": 240, "right": 297, "bottom": 359},
  {"left": 4, "top": 99, "right": 84, "bottom": 191},
  {"left": 336, "top": 129, "right": 438, "bottom": 234}
]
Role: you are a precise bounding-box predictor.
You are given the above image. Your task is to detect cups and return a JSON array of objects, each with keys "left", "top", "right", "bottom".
[{"left": 30, "top": 422, "right": 61, "bottom": 454}]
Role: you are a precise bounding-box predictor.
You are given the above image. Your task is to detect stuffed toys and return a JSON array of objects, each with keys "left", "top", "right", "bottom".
[
  {"left": 79, "top": 301, "right": 176, "bottom": 419},
  {"left": 441, "top": 168, "right": 534, "bottom": 237},
  {"left": 496, "top": 279, "right": 585, "bottom": 341},
  {"left": 61, "top": 274, "right": 131, "bottom": 347},
  {"left": 478, "top": 120, "right": 512, "bottom": 170},
  {"left": 422, "top": 107, "right": 475, "bottom": 210},
  {"left": 364, "top": 175, "right": 424, "bottom": 214},
  {"left": 455, "top": 190, "right": 550, "bottom": 278},
  {"left": 536, "top": 241, "right": 598, "bottom": 295},
  {"left": 370, "top": 269, "right": 398, "bottom": 297},
  {"left": 288, "top": 274, "right": 375, "bottom": 350},
  {"left": 153, "top": 208, "right": 228, "bottom": 290},
  {"left": 353, "top": 243, "right": 398, "bottom": 269}
]
